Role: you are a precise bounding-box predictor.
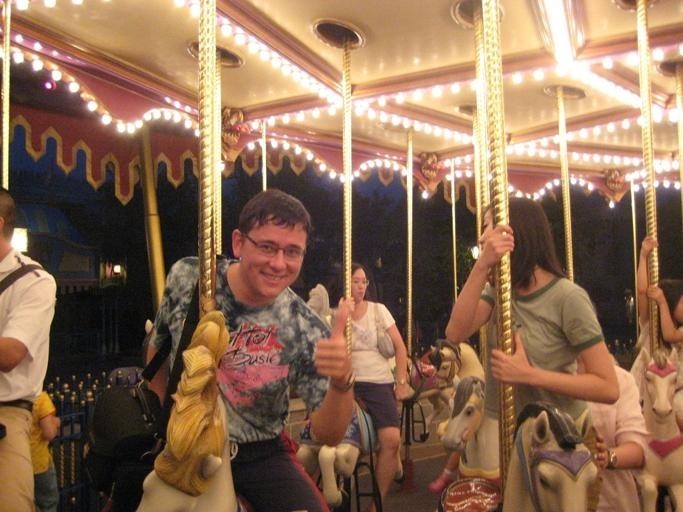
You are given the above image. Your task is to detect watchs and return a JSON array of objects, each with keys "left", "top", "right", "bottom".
[
  {"left": 606, "top": 450, "right": 617, "bottom": 471},
  {"left": 331, "top": 371, "right": 354, "bottom": 393},
  {"left": 396, "top": 379, "right": 406, "bottom": 386}
]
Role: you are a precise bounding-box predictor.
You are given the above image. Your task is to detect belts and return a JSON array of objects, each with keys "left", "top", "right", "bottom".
[{"left": 1, "top": 399, "right": 34, "bottom": 412}]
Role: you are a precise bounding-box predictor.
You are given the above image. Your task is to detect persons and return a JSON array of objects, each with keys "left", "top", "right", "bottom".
[
  {"left": 444, "top": 198, "right": 623, "bottom": 473},
  {"left": 636, "top": 236, "right": 682, "bottom": 430},
  {"left": 31, "top": 357, "right": 63, "bottom": 512},
  {"left": 113, "top": 189, "right": 355, "bottom": 511},
  {"left": 330, "top": 264, "right": 416, "bottom": 511},
  {"left": 426, "top": 450, "right": 460, "bottom": 493},
  {"left": 574, "top": 349, "right": 650, "bottom": 511},
  {"left": 80, "top": 386, "right": 146, "bottom": 511},
  {"left": 1, "top": 186, "right": 58, "bottom": 512}
]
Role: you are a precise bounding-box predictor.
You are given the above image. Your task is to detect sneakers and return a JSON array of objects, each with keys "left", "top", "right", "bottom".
[{"left": 428, "top": 473, "right": 453, "bottom": 494}]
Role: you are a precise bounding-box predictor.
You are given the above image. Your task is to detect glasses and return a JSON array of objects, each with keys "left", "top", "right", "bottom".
[
  {"left": 245, "top": 234, "right": 302, "bottom": 259},
  {"left": 353, "top": 278, "right": 369, "bottom": 286}
]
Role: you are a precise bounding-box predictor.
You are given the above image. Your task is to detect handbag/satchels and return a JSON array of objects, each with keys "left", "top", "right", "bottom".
[
  {"left": 377, "top": 331, "right": 395, "bottom": 359},
  {"left": 91, "top": 385, "right": 165, "bottom": 471}
]
